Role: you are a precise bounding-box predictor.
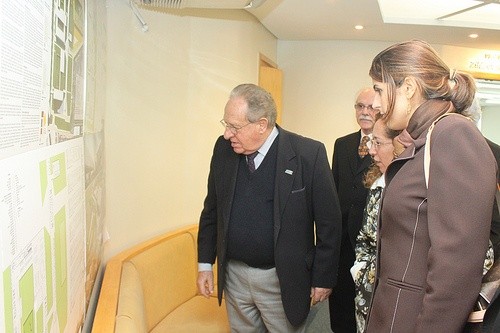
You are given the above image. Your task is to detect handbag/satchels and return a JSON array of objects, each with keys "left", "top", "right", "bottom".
[{"left": 468, "top": 258, "right": 500, "bottom": 322}]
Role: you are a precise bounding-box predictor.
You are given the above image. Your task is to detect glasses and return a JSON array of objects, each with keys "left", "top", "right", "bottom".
[
  {"left": 372, "top": 141, "right": 392, "bottom": 148},
  {"left": 220, "top": 119, "right": 252, "bottom": 133},
  {"left": 354, "top": 104, "right": 373, "bottom": 111}
]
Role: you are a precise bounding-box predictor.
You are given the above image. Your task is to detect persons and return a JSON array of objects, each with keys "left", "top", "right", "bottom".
[
  {"left": 327, "top": 39, "right": 500, "bottom": 332},
  {"left": 197, "top": 84, "right": 342, "bottom": 333}
]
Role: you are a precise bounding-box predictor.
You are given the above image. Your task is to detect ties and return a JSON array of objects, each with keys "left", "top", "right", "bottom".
[
  {"left": 247, "top": 151, "right": 259, "bottom": 175},
  {"left": 358, "top": 136, "right": 370, "bottom": 159}
]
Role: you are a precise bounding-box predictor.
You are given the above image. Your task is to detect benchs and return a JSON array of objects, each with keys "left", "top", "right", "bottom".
[{"left": 91, "top": 223, "right": 230, "bottom": 333}]
